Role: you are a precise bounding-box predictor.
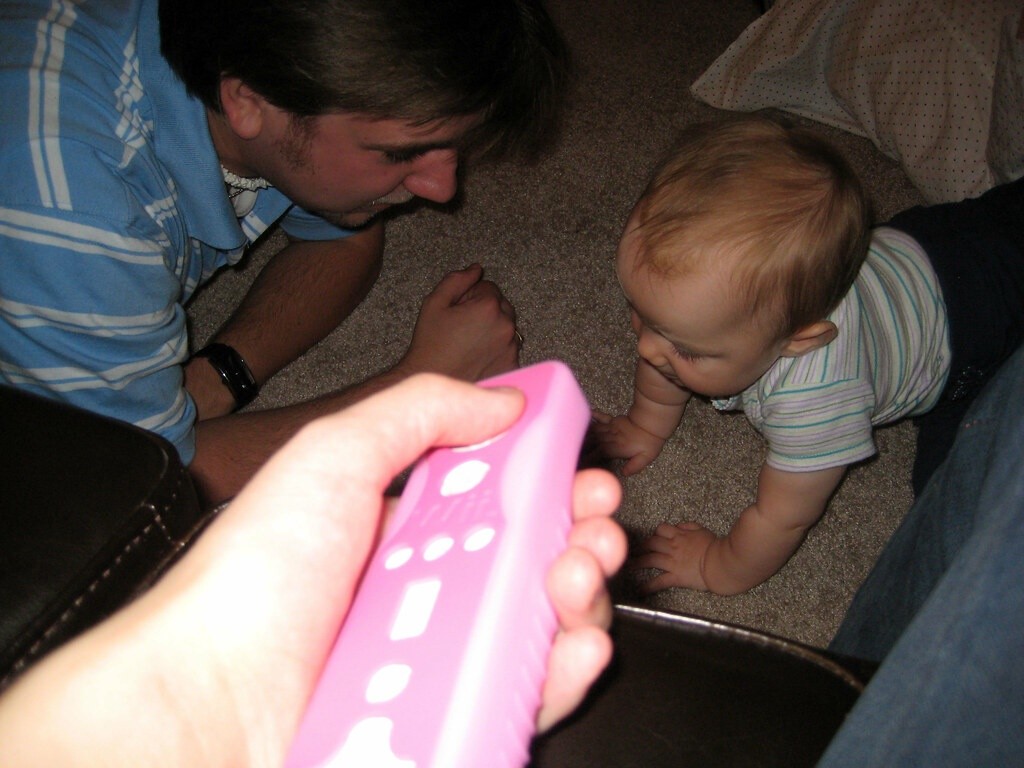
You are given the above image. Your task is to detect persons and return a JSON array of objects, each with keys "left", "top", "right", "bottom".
[
  {"left": 0, "top": 0, "right": 567, "bottom": 505},
  {"left": 581, "top": 116, "right": 1024, "bottom": 596},
  {"left": 0, "top": 350, "right": 1024, "bottom": 768}
]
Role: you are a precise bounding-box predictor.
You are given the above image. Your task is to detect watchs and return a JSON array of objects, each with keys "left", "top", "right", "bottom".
[{"left": 191, "top": 344, "right": 259, "bottom": 414}]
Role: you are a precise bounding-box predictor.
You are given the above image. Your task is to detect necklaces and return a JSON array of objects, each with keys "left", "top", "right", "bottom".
[{"left": 221, "top": 166, "right": 274, "bottom": 198}]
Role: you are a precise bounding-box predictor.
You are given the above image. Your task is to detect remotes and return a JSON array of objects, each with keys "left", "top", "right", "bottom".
[{"left": 279, "top": 360, "right": 591, "bottom": 768}]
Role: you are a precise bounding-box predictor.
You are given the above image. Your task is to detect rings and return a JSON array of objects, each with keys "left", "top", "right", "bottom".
[{"left": 515, "top": 330, "right": 524, "bottom": 344}]
